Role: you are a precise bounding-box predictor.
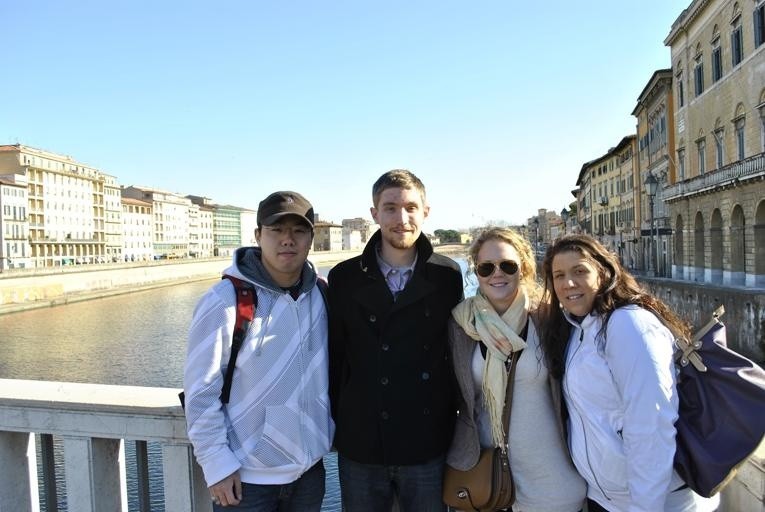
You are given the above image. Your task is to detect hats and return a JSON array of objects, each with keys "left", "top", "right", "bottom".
[{"left": 257, "top": 192, "right": 313, "bottom": 230}]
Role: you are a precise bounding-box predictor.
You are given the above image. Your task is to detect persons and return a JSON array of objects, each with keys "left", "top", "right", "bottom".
[
  {"left": 326, "top": 170, "right": 465, "bottom": 512},
  {"left": 445, "top": 227, "right": 588, "bottom": 512},
  {"left": 534, "top": 232, "right": 721, "bottom": 512},
  {"left": 185, "top": 191, "right": 336, "bottom": 512}
]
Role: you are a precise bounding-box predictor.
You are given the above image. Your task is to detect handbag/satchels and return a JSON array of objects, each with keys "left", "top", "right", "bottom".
[
  {"left": 442, "top": 447, "right": 515, "bottom": 511},
  {"left": 672, "top": 315, "right": 765, "bottom": 497}
]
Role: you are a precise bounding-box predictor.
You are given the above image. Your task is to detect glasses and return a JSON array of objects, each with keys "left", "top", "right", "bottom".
[{"left": 477, "top": 261, "right": 518, "bottom": 277}]
